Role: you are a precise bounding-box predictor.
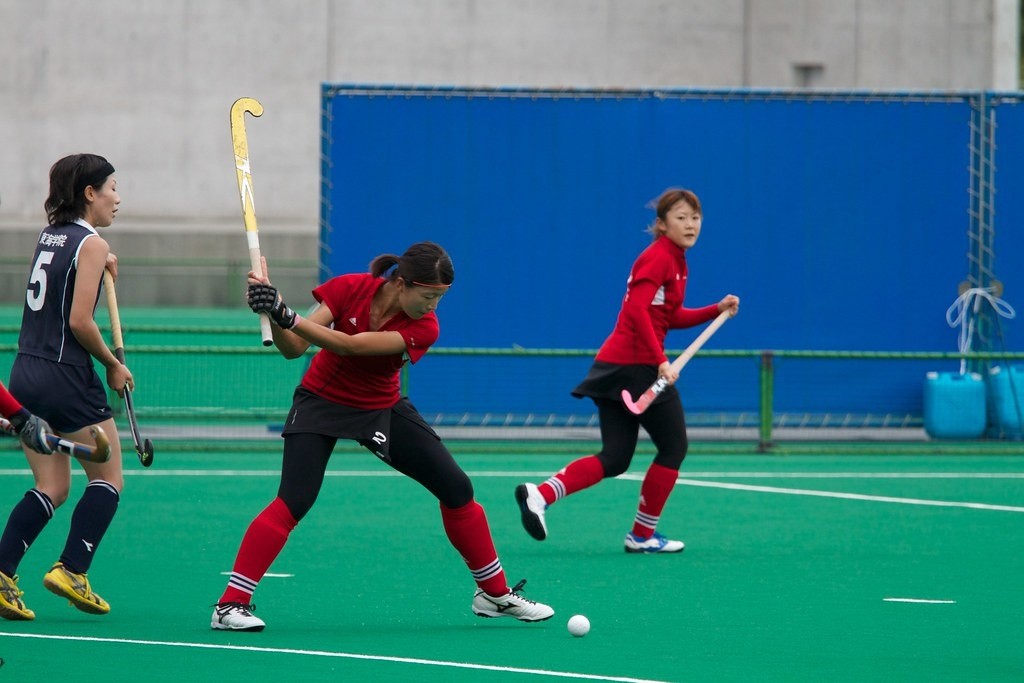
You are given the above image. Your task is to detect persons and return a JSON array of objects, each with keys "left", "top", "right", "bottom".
[
  {"left": 0, "top": 154, "right": 135, "bottom": 620},
  {"left": 0, "top": 379, "right": 55, "bottom": 456},
  {"left": 515, "top": 190, "right": 739, "bottom": 552},
  {"left": 211, "top": 242, "right": 555, "bottom": 631}
]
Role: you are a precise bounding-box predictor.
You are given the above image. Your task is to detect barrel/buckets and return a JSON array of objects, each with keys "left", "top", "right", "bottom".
[{"left": 923, "top": 366, "right": 1024, "bottom": 441}]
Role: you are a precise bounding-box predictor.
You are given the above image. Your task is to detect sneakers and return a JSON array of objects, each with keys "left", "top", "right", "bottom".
[
  {"left": 209, "top": 599, "right": 265, "bottom": 630},
  {"left": 43, "top": 562, "right": 110, "bottom": 614},
  {"left": 625, "top": 531, "right": 684, "bottom": 552},
  {"left": 0, "top": 571, "right": 35, "bottom": 620},
  {"left": 515, "top": 483, "right": 548, "bottom": 541},
  {"left": 472, "top": 579, "right": 554, "bottom": 622}
]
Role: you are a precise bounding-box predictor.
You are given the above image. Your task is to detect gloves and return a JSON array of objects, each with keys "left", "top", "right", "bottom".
[
  {"left": 245, "top": 284, "right": 301, "bottom": 330},
  {"left": 8, "top": 407, "right": 55, "bottom": 455}
]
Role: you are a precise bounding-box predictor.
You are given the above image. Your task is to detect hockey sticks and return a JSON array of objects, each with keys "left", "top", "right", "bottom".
[
  {"left": 0, "top": 414, "right": 113, "bottom": 466},
  {"left": 103, "top": 262, "right": 157, "bottom": 469},
  {"left": 229, "top": 95, "right": 277, "bottom": 348},
  {"left": 619, "top": 308, "right": 732, "bottom": 418}
]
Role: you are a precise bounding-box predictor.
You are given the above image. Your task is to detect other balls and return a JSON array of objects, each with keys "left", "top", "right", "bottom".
[{"left": 565, "top": 614, "right": 592, "bottom": 638}]
